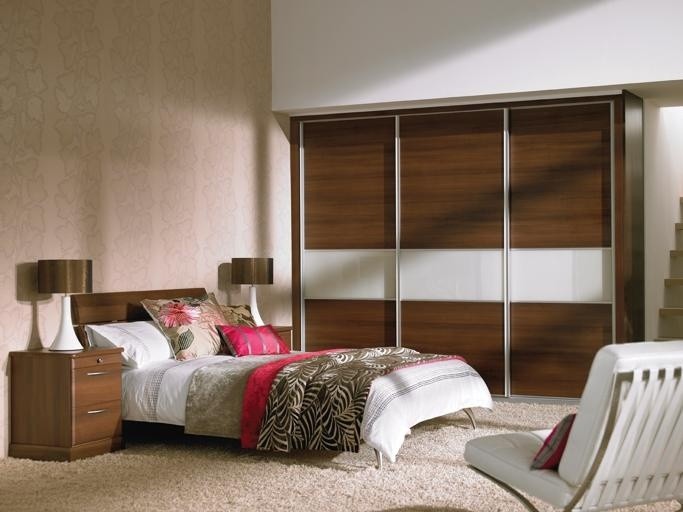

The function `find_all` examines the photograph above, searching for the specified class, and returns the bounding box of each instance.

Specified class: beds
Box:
[71,288,476,469]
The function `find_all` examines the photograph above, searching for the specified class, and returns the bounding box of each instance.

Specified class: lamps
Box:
[231,258,273,326]
[37,259,92,351]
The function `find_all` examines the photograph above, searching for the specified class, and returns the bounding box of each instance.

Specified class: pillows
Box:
[530,412,576,470]
[84,291,290,369]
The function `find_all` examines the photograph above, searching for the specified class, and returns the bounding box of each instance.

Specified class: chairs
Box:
[464,340,683,512]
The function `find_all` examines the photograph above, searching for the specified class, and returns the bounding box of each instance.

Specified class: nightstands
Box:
[9,347,125,463]
